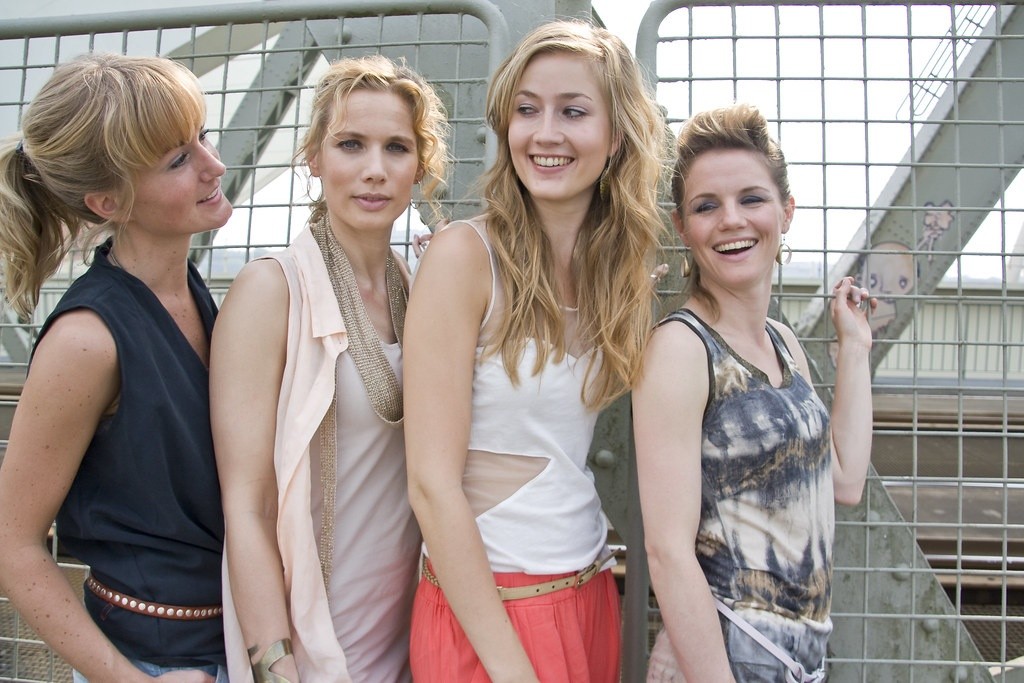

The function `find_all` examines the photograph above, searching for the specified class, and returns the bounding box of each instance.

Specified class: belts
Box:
[712,597,827,683]
[422,548,623,601]
[86,574,224,621]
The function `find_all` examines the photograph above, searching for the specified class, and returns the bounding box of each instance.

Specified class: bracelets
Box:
[247,639,294,683]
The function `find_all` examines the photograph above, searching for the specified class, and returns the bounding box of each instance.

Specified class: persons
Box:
[404,22,661,683]
[0,53,233,683]
[631,107,877,683]
[207,58,444,683]
